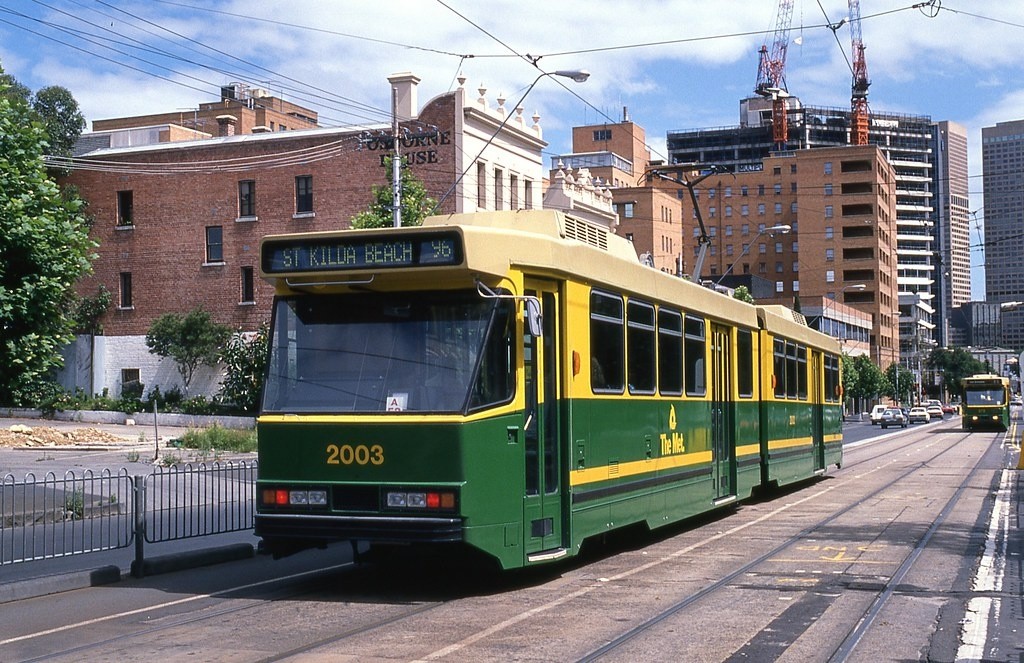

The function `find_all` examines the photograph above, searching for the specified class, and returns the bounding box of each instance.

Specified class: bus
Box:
[959,372,1011,432]
[252,161,843,571]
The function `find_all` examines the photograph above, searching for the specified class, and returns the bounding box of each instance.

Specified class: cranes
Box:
[753,0,794,152]
[848,0,872,146]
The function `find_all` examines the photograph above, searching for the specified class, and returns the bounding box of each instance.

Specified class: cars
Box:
[869,399,959,428]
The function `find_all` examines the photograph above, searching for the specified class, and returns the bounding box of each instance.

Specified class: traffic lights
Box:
[915,392,919,406]
